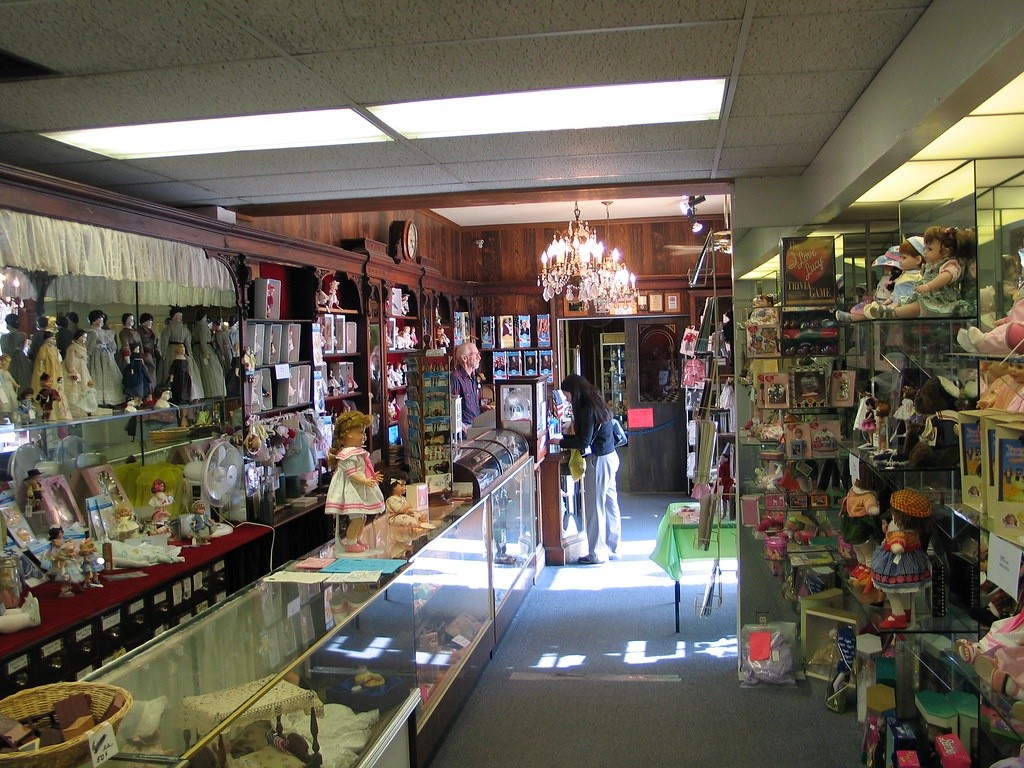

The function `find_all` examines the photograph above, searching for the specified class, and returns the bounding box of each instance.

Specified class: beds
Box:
[180,673,323,768]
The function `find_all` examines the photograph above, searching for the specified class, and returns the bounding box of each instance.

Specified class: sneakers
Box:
[578,556,592,564]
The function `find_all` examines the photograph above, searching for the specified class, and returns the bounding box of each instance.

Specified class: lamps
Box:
[679,195,709,233]
[536,201,639,305]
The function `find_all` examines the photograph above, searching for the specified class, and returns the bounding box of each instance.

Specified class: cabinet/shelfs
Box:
[600,332,627,431]
[316,306,363,402]
[738,301,1024,768]
[383,311,420,428]
[687,289,738,500]
[246,318,312,414]
[480,347,554,387]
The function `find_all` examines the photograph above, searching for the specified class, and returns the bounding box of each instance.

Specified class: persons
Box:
[435,307,478,348]
[743,294,778,324]
[713,453,734,519]
[835,226,1024,457]
[483,317,551,412]
[605,349,627,415]
[547,374,619,564]
[491,487,517,563]
[0,280,427,598]
[967,447,1024,528]
[839,466,933,629]
[450,342,495,439]
[832,621,856,710]
[957,606,1024,721]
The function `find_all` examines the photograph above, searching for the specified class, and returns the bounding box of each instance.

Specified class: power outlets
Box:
[756,610,770,623]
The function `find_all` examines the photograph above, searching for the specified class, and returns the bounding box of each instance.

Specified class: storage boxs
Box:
[887,717,916,768]
[800,588,843,665]
[806,607,861,688]
[455,311,554,384]
[775,235,836,304]
[936,733,972,768]
[895,749,920,768]
[39,278,357,535]
[914,688,959,768]
[946,689,979,768]
[405,482,429,511]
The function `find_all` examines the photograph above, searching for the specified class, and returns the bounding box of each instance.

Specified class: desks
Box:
[650,500,740,632]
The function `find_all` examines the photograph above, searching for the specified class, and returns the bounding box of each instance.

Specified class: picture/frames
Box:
[563,293,590,319]
[595,304,609,313]
[665,289,684,316]
[647,292,665,315]
[638,294,648,312]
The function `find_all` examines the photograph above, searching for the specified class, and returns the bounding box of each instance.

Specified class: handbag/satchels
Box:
[579,444,592,457]
[610,418,627,447]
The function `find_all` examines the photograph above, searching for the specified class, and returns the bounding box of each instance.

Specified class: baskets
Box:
[1,681,131,768]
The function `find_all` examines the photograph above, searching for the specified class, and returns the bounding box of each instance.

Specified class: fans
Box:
[183,439,246,538]
[7,444,63,475]
[58,436,105,472]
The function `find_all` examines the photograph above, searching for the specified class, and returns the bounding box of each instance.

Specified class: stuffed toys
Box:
[872,376,979,471]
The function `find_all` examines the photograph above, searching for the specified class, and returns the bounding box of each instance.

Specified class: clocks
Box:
[402,218,418,262]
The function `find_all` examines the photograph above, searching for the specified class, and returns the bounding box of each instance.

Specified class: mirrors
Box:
[0,274,241,541]
[762,156,1024,716]
